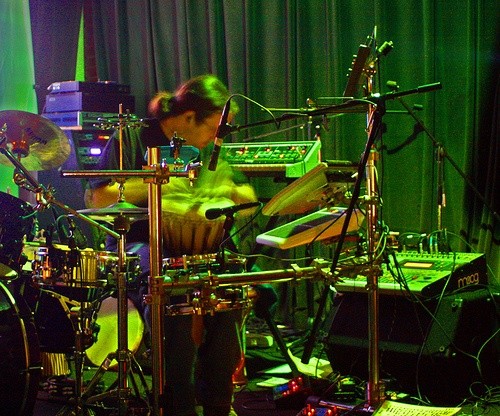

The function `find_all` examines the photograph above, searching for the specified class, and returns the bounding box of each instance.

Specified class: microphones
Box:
[205,202,261,220]
[208,101,231,171]
[343,45,370,103]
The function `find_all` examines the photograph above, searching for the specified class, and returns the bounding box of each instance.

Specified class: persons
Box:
[84,75,260,416]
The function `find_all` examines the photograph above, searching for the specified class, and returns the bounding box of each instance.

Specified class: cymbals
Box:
[67,203,148,224]
[50,374,105,397]
[0,110,70,171]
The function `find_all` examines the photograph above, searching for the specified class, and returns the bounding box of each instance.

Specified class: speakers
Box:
[326,286,500,407]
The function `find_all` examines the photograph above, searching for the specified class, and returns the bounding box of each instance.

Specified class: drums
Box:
[0,190,35,281]
[84,296,145,370]
[0,281,42,416]
[160,192,236,256]
[33,246,143,290]
[162,254,252,316]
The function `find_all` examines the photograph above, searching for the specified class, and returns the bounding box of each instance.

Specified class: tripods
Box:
[68,215,155,416]
